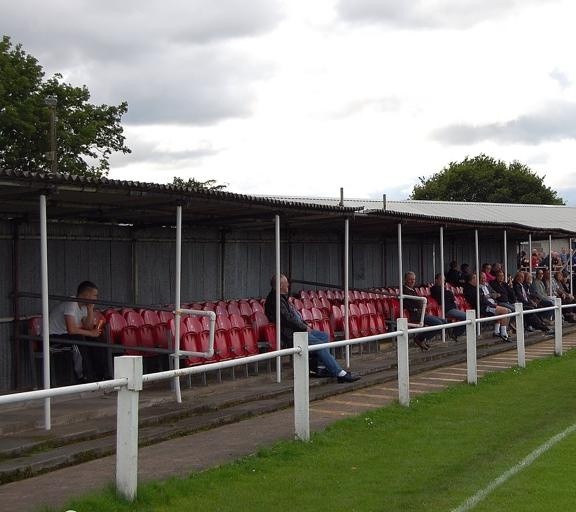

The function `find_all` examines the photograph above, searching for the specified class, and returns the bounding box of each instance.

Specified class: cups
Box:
[93,320,104,329]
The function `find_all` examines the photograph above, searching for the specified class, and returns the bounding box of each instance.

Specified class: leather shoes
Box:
[526,327,534,332]
[415,337,428,350]
[451,336,457,342]
[416,335,431,348]
[336,372,360,383]
[310,367,333,378]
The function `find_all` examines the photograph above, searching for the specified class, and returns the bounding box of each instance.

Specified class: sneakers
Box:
[493,332,511,338]
[544,329,555,337]
[500,335,512,344]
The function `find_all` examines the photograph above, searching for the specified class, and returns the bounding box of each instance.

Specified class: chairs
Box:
[25,283,505,390]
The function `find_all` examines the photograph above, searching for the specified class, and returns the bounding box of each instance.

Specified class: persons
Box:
[264,273,361,384]
[38,280,103,384]
[446,247,576,343]
[402,270,446,350]
[430,272,466,342]
